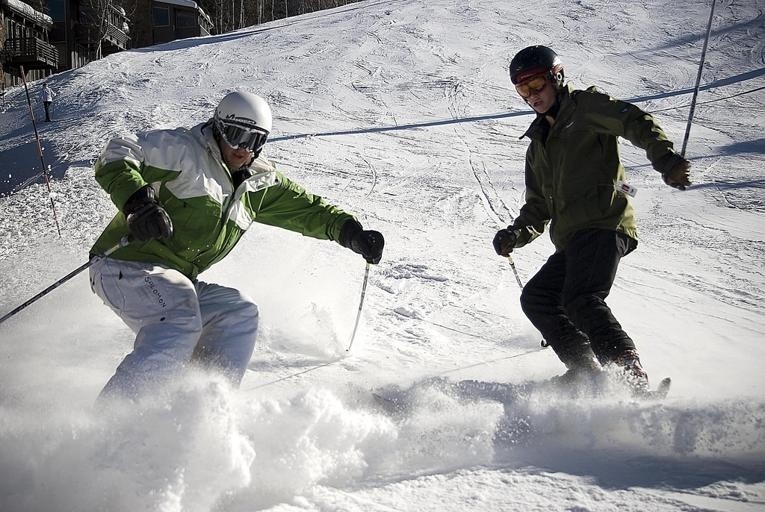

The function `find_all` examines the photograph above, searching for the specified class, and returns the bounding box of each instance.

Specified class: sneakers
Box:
[608,363,650,386]
[547,358,602,384]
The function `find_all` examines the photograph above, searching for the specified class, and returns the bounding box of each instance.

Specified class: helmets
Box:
[508,45,565,82]
[215,91,273,134]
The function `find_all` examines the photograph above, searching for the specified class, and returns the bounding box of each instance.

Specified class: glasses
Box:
[513,71,551,99]
[215,119,267,152]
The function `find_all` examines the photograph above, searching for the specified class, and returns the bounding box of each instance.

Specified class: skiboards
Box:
[371,378,672,432]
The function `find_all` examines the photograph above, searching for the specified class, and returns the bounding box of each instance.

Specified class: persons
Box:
[83,89,387,402]
[490,44,695,400]
[36,82,57,122]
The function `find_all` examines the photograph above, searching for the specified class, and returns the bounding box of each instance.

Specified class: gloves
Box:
[122,185,174,241]
[493,228,516,258]
[340,216,384,265]
[652,149,693,190]
